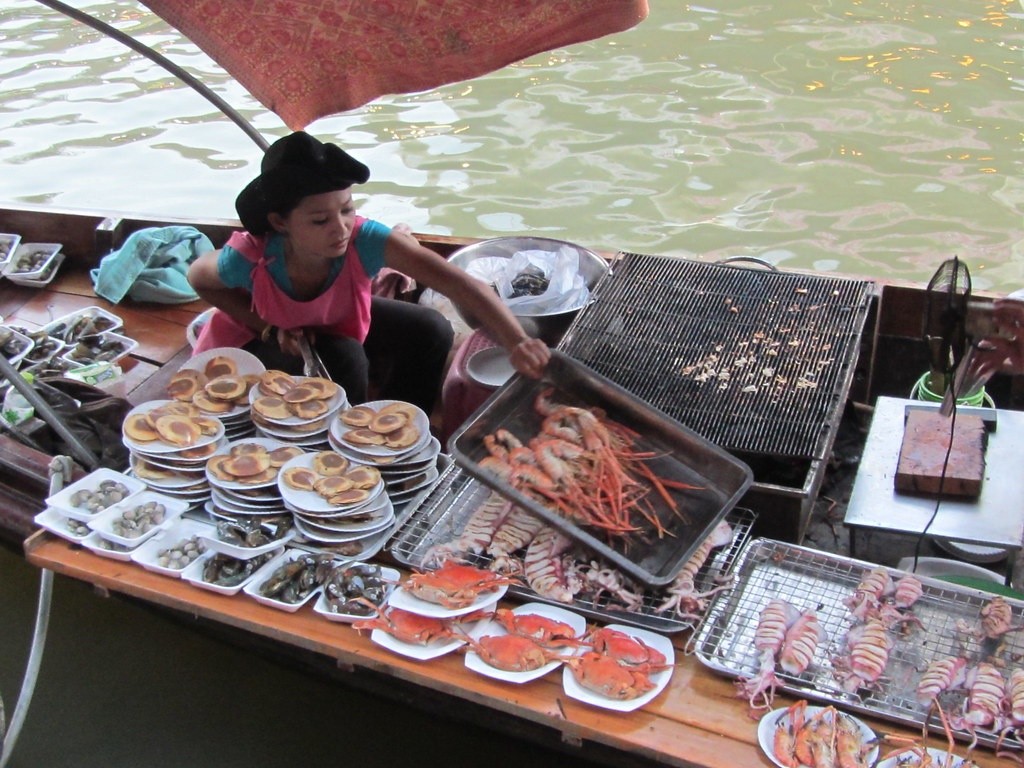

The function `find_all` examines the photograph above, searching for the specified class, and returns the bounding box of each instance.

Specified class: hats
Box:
[234,127,370,236]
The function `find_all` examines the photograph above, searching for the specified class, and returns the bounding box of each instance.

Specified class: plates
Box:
[758,706,879,768]
[879,745,980,766]
[369,566,676,712]
[121,345,442,542]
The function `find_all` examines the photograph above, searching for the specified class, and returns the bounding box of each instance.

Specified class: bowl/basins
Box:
[33,466,401,630]
[438,237,611,343]
[0,231,139,392]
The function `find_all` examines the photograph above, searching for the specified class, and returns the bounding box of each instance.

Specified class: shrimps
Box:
[480,388,707,556]
[773,699,978,768]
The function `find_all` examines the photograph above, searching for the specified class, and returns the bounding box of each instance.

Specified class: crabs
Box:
[346,562,685,701]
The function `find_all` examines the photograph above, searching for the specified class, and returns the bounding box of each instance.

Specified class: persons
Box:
[187,131,552,417]
